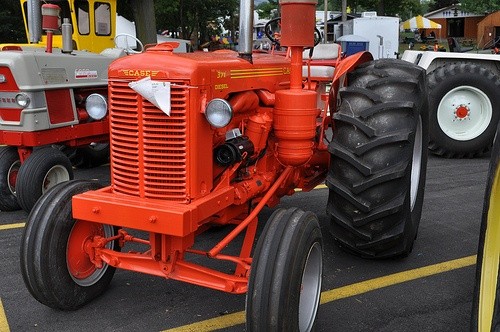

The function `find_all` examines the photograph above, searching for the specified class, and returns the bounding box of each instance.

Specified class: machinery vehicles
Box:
[0,0,158,213]
[18,0,431,332]
[400,50,500,159]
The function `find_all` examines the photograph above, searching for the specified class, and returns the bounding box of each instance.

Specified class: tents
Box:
[400,15,442,46]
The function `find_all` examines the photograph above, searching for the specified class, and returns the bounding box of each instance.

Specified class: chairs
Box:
[301,43,342,99]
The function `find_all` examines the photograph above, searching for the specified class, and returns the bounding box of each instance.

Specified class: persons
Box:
[264,41,271,50]
[447,38,456,51]
[420,31,425,43]
[430,31,435,37]
